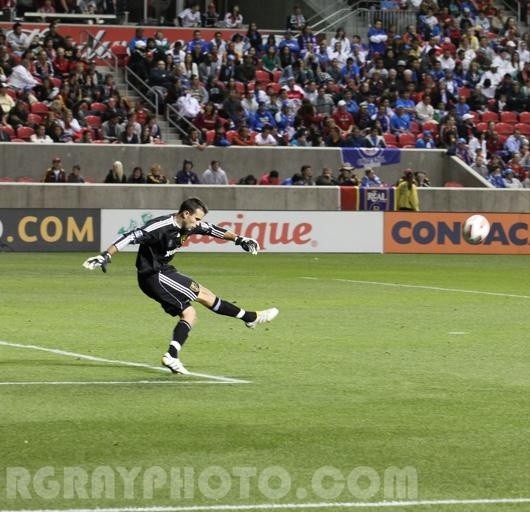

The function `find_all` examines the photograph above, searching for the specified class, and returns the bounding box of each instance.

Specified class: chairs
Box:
[224,131,238,145]
[0,126,16,141]
[382,132,396,144]
[205,131,215,144]
[515,123,530,134]
[16,127,35,140]
[255,70,270,82]
[416,132,424,140]
[467,111,482,123]
[51,79,61,86]
[486,100,497,110]
[402,143,416,148]
[475,122,488,132]
[423,119,441,137]
[76,128,96,142]
[85,115,101,129]
[482,112,501,122]
[398,131,416,147]
[91,102,107,114]
[234,81,246,98]
[268,82,280,95]
[6,88,18,100]
[386,143,398,148]
[444,182,464,188]
[408,122,420,134]
[16,176,31,182]
[248,82,257,93]
[274,71,282,83]
[0,176,16,183]
[258,81,268,92]
[519,110,530,123]
[111,44,129,66]
[9,139,25,144]
[31,101,48,115]
[494,123,514,134]
[458,88,470,99]
[92,138,110,144]
[27,113,43,126]
[499,110,517,123]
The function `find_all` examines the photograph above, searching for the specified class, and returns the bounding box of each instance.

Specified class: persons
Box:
[41,157,66,183]
[318,166,337,185]
[176,159,200,183]
[395,169,420,211]
[337,163,358,186]
[147,163,170,184]
[367,56,389,151]
[0,0,107,24]
[175,4,201,26]
[103,161,127,182]
[390,41,440,148]
[286,5,306,30]
[115,27,367,149]
[67,165,84,182]
[82,197,279,374]
[368,21,387,55]
[204,3,220,27]
[441,33,530,116]
[225,6,243,27]
[345,0,520,38]
[439,113,530,187]
[127,167,144,183]
[201,160,228,184]
[238,174,257,185]
[0,23,114,144]
[258,170,282,185]
[360,166,385,187]
[301,165,316,185]
[282,173,304,185]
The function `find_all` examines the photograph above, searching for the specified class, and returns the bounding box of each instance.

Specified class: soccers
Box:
[462,214,491,246]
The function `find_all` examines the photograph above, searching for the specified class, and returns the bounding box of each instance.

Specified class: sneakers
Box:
[163,353,190,375]
[247,308,280,327]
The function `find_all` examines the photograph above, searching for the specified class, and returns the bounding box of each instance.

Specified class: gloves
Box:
[235,235,260,256]
[82,251,113,273]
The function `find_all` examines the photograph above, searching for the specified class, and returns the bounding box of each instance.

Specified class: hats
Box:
[52,156,62,163]
[463,113,476,120]
[425,131,432,137]
[503,169,512,175]
[395,60,408,67]
[281,101,294,109]
[338,162,355,172]
[404,168,414,174]
[338,100,348,107]
[242,50,254,59]
